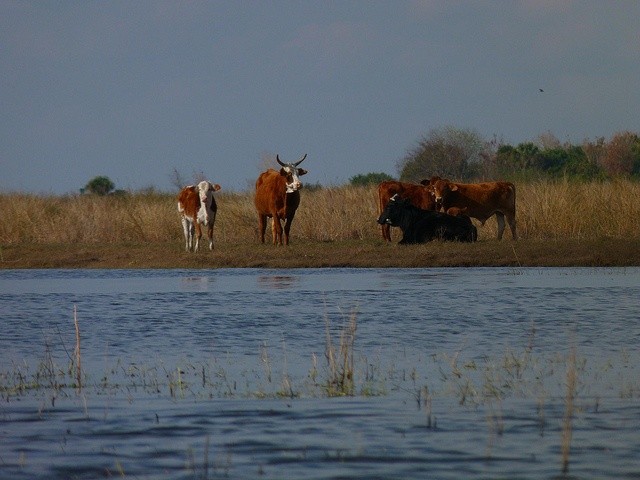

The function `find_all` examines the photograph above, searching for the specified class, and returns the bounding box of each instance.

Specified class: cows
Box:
[431,178,520,242]
[376,174,450,243]
[253,153,309,250]
[376,192,478,246]
[176,179,222,254]
